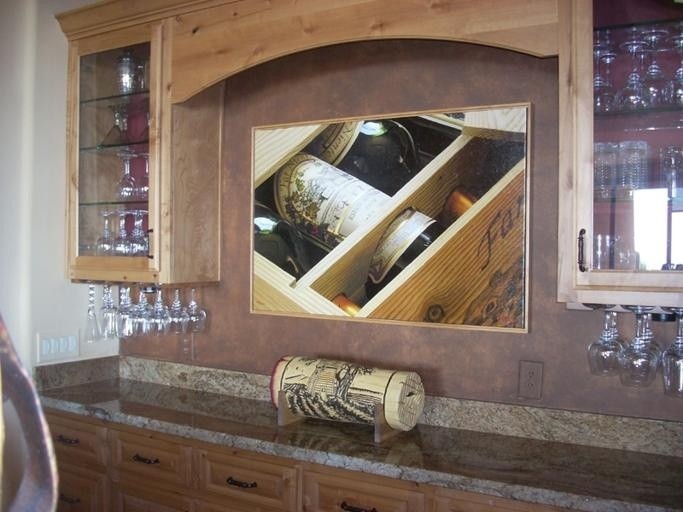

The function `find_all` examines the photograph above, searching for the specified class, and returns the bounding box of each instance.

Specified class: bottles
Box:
[260,156,444,281]
[348,122,477,224]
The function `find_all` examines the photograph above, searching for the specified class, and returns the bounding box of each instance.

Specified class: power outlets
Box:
[516,359,542,399]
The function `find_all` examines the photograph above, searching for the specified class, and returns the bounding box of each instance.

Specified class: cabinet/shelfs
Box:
[556,1,683,308]
[66,16,221,284]
[39,404,568,512]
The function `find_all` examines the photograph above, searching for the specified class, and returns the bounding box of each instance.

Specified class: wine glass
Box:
[583,304,683,397]
[82,282,207,343]
[591,29,682,113]
[96,152,147,254]
[100,102,138,147]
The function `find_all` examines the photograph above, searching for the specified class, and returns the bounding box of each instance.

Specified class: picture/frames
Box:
[247,101,533,335]
